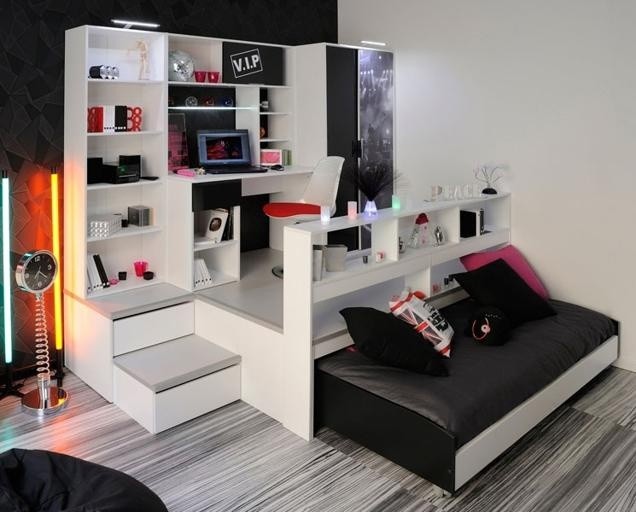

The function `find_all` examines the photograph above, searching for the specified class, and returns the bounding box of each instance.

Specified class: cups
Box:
[207,71,220,83]
[195,71,207,85]
[134,261,147,277]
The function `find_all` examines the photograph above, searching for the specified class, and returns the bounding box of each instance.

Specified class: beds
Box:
[283,188,620,494]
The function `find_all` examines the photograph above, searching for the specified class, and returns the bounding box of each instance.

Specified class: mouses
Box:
[271,165,284,171]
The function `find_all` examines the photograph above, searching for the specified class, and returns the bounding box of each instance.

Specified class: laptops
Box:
[196,128,268,174]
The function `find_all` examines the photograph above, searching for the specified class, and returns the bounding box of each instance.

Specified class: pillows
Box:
[338,307,457,377]
[460,244,549,299]
[388,290,455,358]
[452,258,557,332]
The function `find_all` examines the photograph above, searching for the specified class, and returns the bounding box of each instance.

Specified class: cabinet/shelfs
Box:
[64,24,395,436]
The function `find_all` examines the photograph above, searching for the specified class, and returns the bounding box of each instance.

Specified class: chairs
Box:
[263,157,345,279]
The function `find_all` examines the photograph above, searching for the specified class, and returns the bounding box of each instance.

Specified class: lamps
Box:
[51,165,65,387]
[361,40,386,46]
[0,167,25,401]
[110,18,160,29]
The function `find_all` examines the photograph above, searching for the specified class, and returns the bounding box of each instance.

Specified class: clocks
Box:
[11,249,58,293]
